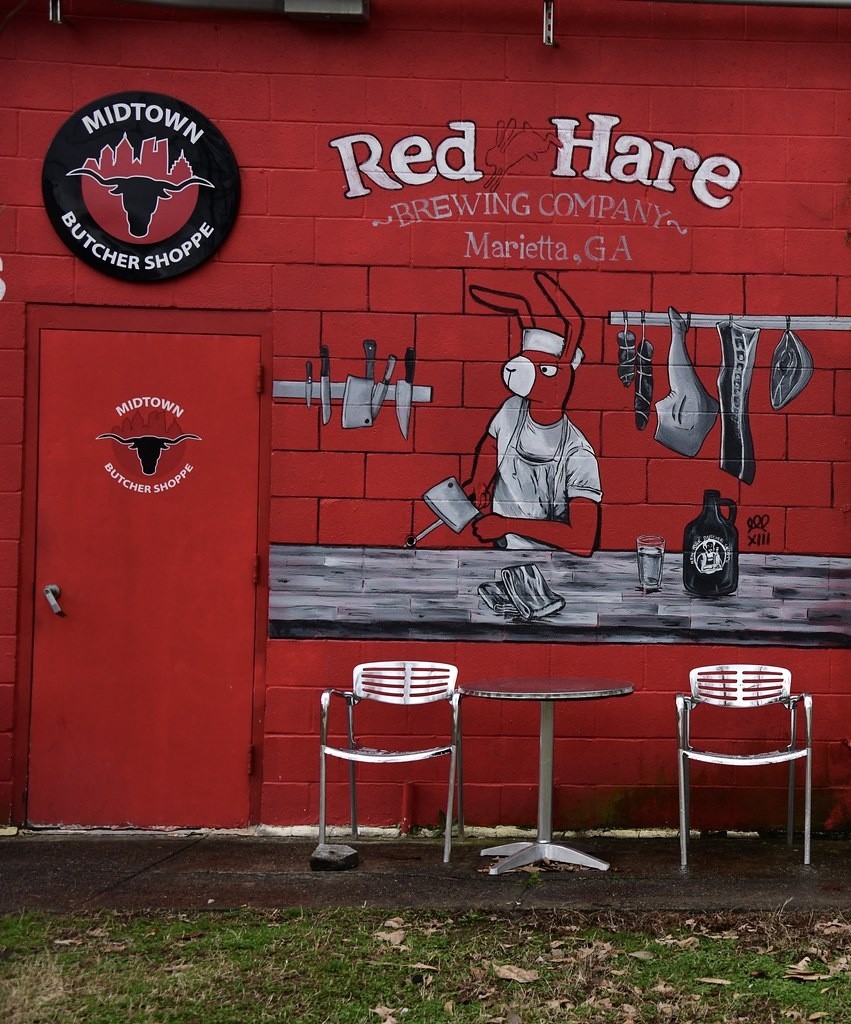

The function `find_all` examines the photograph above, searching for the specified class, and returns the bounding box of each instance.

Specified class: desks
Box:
[457,676,635,876]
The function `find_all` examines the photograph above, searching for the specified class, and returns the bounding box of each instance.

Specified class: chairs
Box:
[316,660,467,864]
[675,663,814,867]
